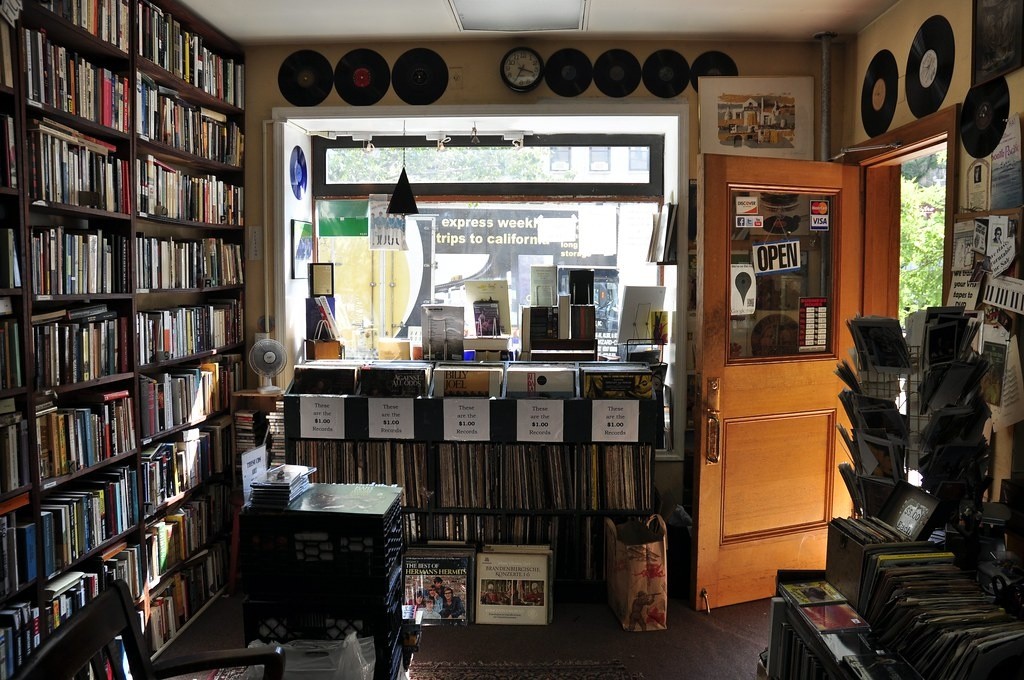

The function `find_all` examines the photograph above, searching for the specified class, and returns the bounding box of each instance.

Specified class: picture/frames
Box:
[696,74,816,163]
[968,0,1024,93]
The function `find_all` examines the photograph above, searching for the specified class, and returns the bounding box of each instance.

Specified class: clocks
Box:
[500,46,544,91]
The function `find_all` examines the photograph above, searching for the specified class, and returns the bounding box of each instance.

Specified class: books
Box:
[238,400,287,486]
[762,528,1024,680]
[292,438,657,627]
[827,304,996,524]
[0,210,249,417]
[291,266,668,396]
[0,0,248,210]
[0,417,234,680]
[647,202,679,265]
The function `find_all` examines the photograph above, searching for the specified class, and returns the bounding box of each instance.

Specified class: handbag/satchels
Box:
[606,513,668,631]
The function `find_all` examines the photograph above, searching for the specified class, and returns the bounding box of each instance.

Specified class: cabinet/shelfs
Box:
[0,0,253,677]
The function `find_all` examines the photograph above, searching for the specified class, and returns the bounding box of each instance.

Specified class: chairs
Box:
[8,577,287,680]
